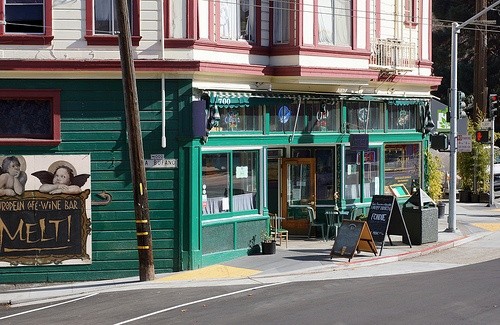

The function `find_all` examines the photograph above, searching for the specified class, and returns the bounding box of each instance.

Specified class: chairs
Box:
[326,209,341,240]
[269,213,289,248]
[306,206,325,241]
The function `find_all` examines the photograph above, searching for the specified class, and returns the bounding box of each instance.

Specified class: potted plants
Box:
[423,147,446,218]
[457,102,500,203]
[261,231,276,254]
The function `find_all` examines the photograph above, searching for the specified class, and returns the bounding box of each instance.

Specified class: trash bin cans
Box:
[403,188,438,244]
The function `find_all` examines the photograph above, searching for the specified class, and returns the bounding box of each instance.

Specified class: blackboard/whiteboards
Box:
[367,195,408,242]
[332,220,377,257]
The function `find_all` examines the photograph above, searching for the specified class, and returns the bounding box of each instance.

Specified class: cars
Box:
[472,145,500,191]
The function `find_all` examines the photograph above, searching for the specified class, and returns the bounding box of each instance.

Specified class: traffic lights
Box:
[458,90,468,119]
[476,130,489,143]
[488,93,499,119]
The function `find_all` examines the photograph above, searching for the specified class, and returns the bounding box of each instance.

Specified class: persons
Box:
[39,165,80,195]
[0,156,26,196]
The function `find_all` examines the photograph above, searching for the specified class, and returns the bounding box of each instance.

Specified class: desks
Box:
[325,211,349,224]
[269,216,286,227]
